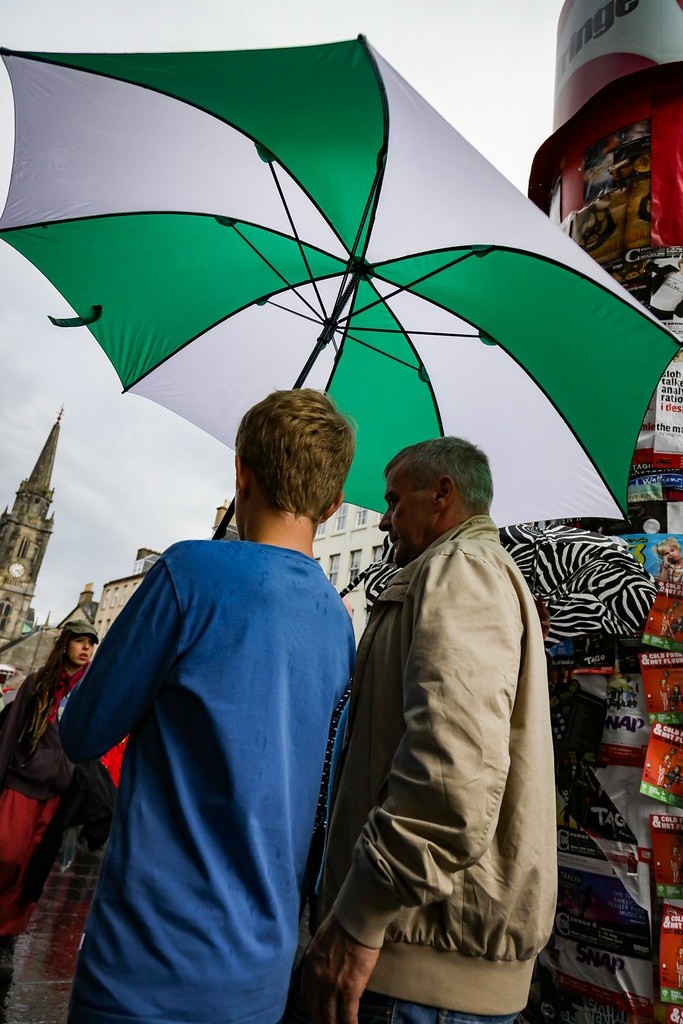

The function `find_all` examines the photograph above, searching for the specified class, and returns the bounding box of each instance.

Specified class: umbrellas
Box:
[0,34,683,540]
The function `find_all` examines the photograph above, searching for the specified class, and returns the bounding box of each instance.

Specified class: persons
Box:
[58,388,357,1024]
[303,436,558,1024]
[0,619,129,976]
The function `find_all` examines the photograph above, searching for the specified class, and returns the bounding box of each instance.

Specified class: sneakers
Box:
[0,936,14,974]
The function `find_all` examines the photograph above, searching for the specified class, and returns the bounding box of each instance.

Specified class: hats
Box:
[63,620,99,644]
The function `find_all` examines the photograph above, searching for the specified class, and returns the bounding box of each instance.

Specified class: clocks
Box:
[7,561,26,579]
